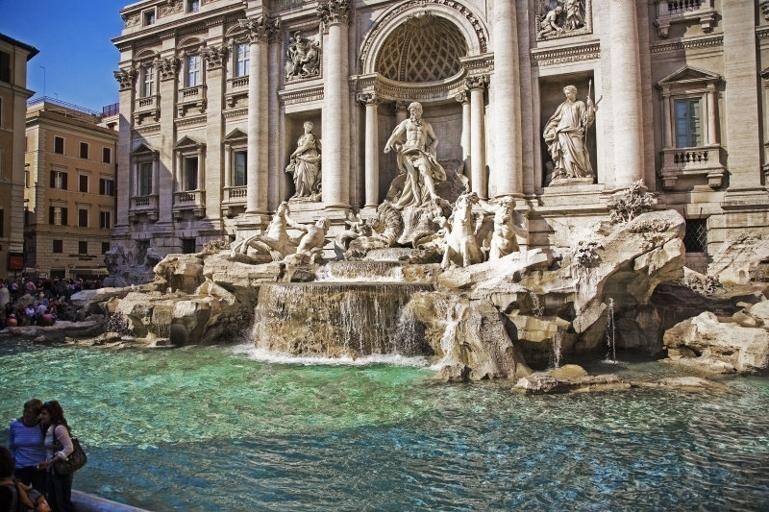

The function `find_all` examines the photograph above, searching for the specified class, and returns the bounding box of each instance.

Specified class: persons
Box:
[37,400,74,512]
[288,35,320,78]
[0,276,83,326]
[0,445,24,512]
[489,195,528,259]
[384,102,440,206]
[537,5,565,38]
[9,399,47,498]
[291,122,318,198]
[285,208,330,264]
[543,85,595,177]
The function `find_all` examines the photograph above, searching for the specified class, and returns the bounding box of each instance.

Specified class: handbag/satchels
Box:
[53,423,88,472]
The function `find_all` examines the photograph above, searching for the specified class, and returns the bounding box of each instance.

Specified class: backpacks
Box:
[0,479,51,512]
